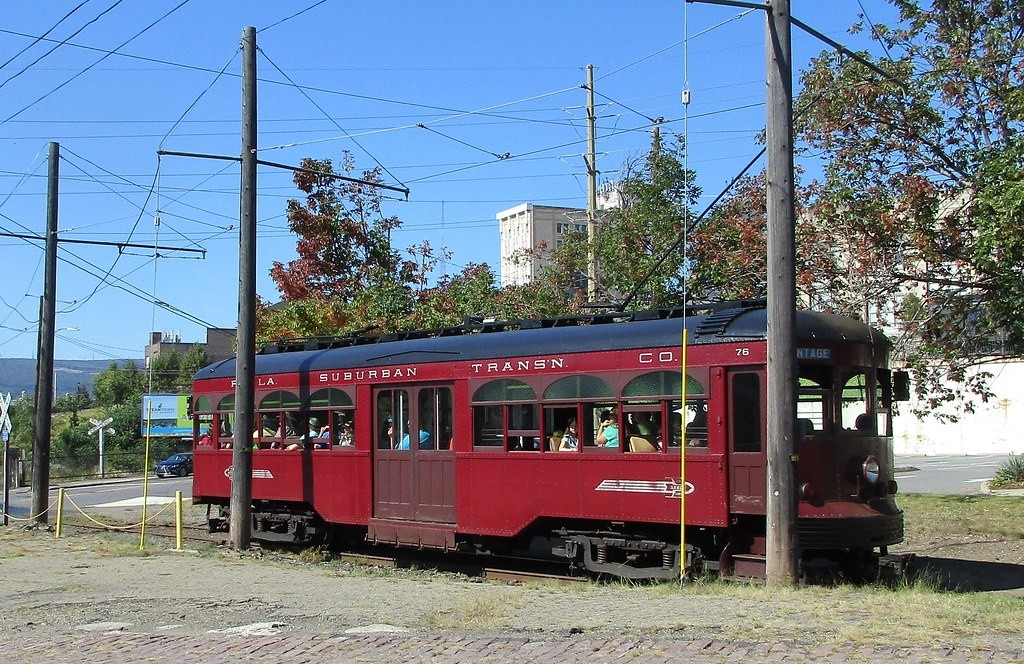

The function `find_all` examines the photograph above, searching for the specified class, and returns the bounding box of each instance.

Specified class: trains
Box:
[193,299,910,585]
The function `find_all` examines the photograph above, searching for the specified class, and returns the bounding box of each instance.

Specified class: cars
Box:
[157,453,193,478]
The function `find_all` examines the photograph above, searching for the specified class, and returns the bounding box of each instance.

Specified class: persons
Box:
[200,420,234,449]
[269,417,354,451]
[387,418,433,450]
[559,416,578,452]
[596,407,661,448]
[252,416,276,449]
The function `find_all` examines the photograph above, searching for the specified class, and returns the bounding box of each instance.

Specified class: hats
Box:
[309,418,320,431]
[276,411,296,421]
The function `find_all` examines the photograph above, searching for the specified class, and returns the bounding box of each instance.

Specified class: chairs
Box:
[626,434,661,453]
[545,435,562,452]
